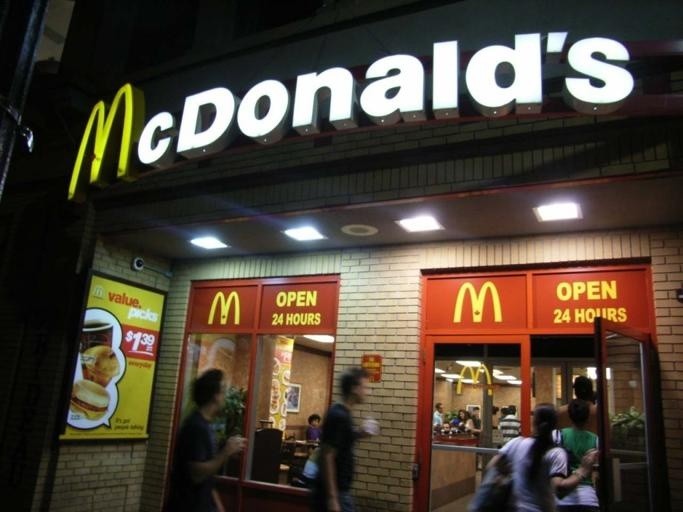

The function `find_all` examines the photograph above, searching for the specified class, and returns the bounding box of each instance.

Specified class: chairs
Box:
[260,419,319,476]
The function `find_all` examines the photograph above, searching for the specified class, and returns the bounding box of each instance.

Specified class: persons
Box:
[160,368,249,511]
[306,368,379,511]
[432,372,601,512]
[302,413,322,484]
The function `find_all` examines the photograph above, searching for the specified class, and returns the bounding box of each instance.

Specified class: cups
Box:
[78,319,114,384]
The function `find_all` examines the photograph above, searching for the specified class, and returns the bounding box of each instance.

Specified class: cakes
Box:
[82,346,120,390]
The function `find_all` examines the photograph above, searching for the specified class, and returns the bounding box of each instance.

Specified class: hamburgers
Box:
[70,379,108,423]
